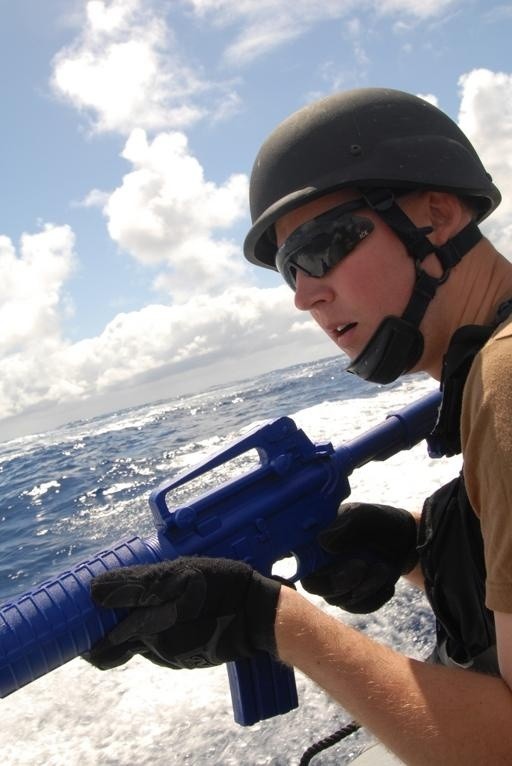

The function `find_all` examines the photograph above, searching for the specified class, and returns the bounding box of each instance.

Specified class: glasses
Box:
[275,192,376,292]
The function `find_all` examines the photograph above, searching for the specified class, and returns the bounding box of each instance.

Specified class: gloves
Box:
[293,502,418,616]
[82,555,281,673]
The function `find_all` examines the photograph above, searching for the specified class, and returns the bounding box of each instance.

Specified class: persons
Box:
[70,84,512,765]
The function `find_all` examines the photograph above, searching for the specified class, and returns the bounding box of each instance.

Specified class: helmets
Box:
[243,88,502,270]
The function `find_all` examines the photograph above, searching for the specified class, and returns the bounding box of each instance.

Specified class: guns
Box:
[0,390,445,725]
